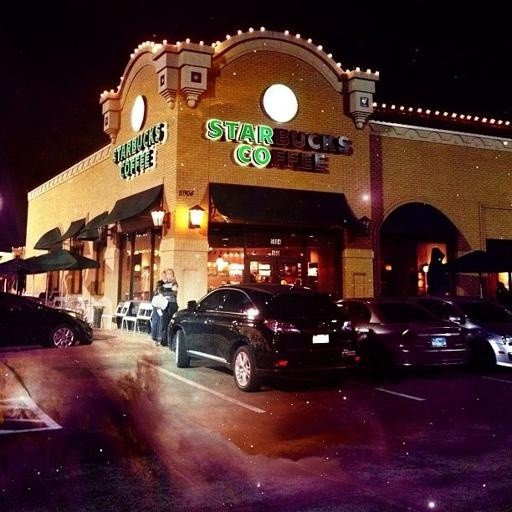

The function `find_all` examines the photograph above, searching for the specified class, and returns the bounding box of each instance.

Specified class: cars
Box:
[1,291,94,348]
[335,296,474,374]
[415,294,512,375]
[167,281,362,393]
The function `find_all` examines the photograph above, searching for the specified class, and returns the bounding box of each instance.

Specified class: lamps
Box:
[187,204,206,230]
[148,203,167,232]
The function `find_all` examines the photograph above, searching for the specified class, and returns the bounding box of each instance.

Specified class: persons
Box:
[150,280,166,346]
[496,282,512,307]
[159,269,179,346]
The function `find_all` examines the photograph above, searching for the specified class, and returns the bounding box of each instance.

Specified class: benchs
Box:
[100,301,154,336]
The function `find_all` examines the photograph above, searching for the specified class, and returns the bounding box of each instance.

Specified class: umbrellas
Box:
[0,248,101,308]
[440,250,512,299]
